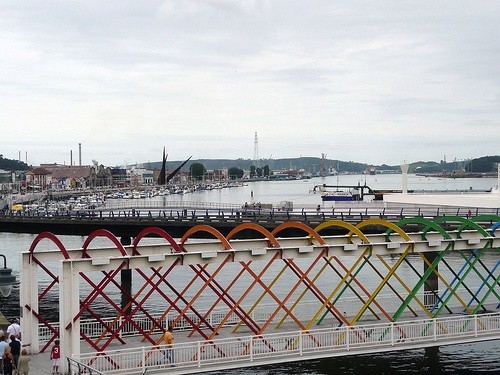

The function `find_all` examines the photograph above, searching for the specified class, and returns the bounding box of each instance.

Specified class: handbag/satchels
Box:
[15,333,20,337]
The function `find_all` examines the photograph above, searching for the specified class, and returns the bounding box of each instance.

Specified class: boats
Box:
[6,182,249,215]
[321,185,355,202]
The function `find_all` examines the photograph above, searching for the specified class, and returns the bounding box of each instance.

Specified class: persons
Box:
[7,319,21,340]
[50,340,60,374]
[0,330,31,375]
[156,326,175,367]
